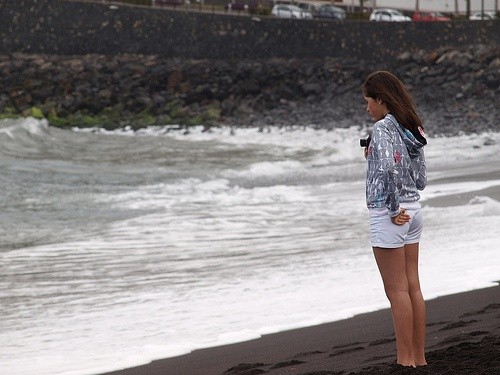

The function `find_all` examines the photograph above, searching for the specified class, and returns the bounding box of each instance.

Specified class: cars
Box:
[369,8,413,21]
[318,4,346,19]
[224,0,259,14]
[469,11,494,20]
[272,4,313,19]
[413,10,451,21]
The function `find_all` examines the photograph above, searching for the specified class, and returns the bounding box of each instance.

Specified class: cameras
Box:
[360,136,372,151]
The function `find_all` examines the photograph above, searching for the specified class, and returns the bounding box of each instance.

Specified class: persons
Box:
[363,71,428,371]
